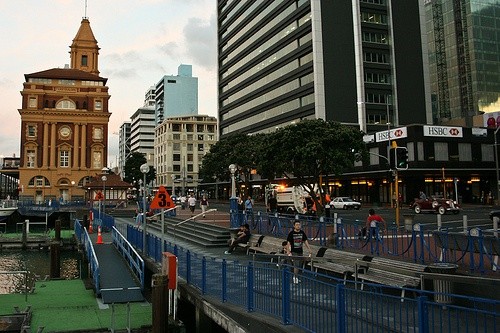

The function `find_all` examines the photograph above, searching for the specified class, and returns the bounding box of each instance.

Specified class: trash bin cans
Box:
[428,263,459,305]
[402,214,415,233]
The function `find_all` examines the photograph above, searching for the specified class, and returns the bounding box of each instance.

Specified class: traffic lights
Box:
[389,147,396,170]
[396,147,408,169]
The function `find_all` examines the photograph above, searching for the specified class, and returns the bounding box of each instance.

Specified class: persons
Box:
[133,209,157,229]
[267,194,278,212]
[420,191,426,200]
[392,191,404,208]
[357,209,387,240]
[276,220,312,284]
[480,189,495,203]
[238,195,255,227]
[179,192,196,217]
[225,224,250,254]
[200,194,209,218]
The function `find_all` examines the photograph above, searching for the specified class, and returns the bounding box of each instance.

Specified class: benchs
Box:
[228,233,426,302]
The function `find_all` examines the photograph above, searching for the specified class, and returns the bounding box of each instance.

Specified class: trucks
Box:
[276,186,331,215]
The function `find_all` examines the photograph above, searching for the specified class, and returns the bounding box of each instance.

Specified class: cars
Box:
[408,194,462,215]
[330,197,361,211]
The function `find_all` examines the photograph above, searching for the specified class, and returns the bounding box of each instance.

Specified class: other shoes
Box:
[293,277,298,284]
[224,250,231,254]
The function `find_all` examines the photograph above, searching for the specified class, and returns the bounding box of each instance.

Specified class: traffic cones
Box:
[88,224,93,234]
[96,225,104,244]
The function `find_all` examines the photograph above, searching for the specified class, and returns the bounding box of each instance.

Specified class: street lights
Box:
[228,163,240,228]
[139,164,149,231]
[171,174,176,194]
[138,179,142,196]
[102,176,107,218]
